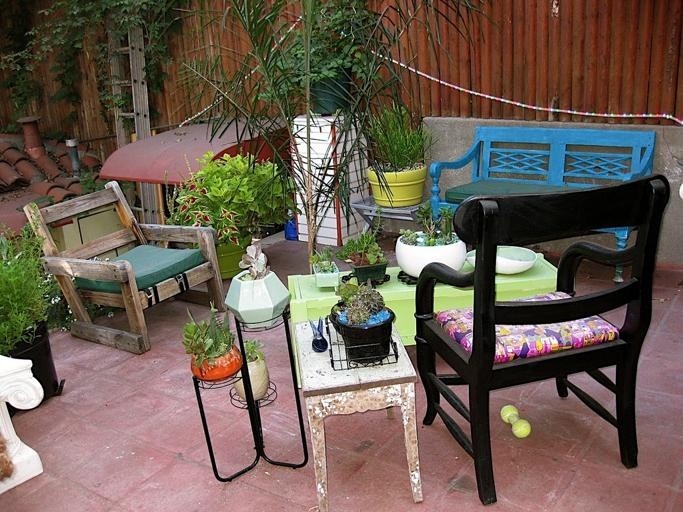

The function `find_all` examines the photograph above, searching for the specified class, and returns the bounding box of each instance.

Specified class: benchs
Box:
[429,125,656,286]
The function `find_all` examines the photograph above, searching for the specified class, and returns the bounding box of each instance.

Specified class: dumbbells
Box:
[501,405,530,439]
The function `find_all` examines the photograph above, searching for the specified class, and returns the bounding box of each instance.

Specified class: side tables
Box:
[295,321,424,512]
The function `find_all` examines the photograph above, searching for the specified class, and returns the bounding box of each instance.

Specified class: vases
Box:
[468,244,537,275]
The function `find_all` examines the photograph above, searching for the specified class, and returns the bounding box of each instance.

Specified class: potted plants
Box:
[181,301,243,382]
[164,152,302,279]
[366,102,427,207]
[334,234,391,288]
[255,1,382,116]
[0,203,65,417]
[329,284,396,363]
[224,239,291,329]
[395,201,467,284]
[234,339,270,402]
[310,247,340,292]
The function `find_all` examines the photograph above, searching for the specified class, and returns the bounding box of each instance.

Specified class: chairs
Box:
[414,174,670,505]
[23,181,225,355]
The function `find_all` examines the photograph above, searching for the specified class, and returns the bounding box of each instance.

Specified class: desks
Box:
[350,194,431,239]
[288,252,558,389]
